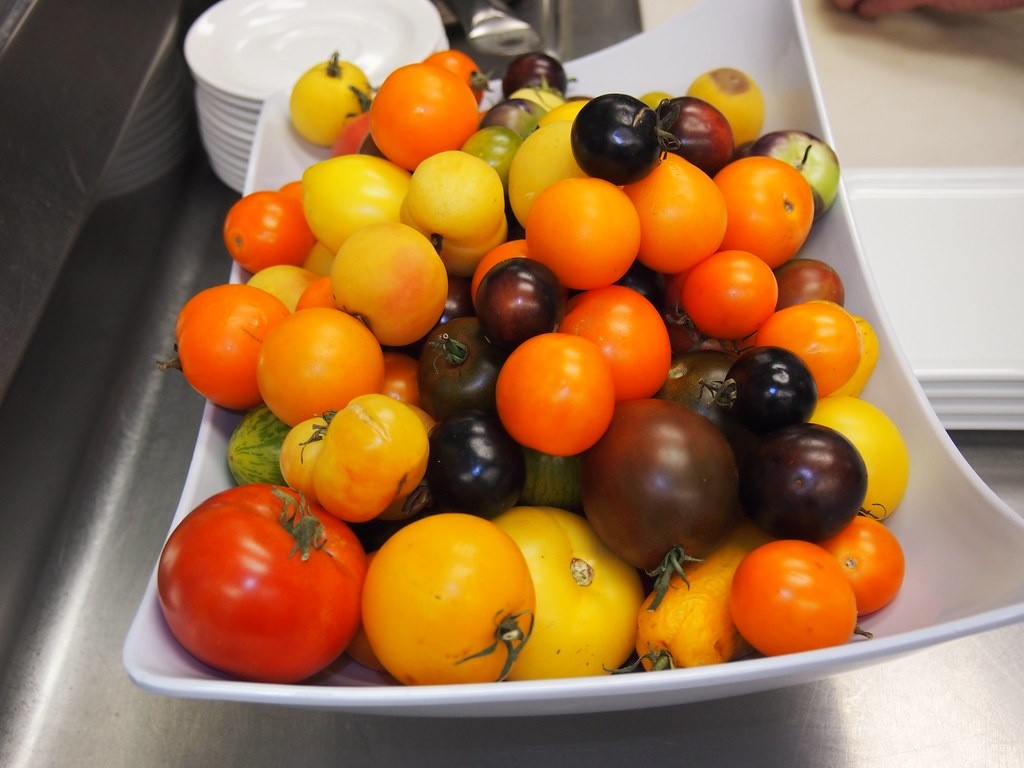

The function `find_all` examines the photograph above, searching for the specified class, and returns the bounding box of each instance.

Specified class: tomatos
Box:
[156,51,910,688]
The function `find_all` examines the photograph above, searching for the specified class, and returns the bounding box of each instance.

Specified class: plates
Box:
[123,0,1024,714]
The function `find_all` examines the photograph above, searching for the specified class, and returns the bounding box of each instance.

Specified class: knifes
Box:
[443,0,542,78]
